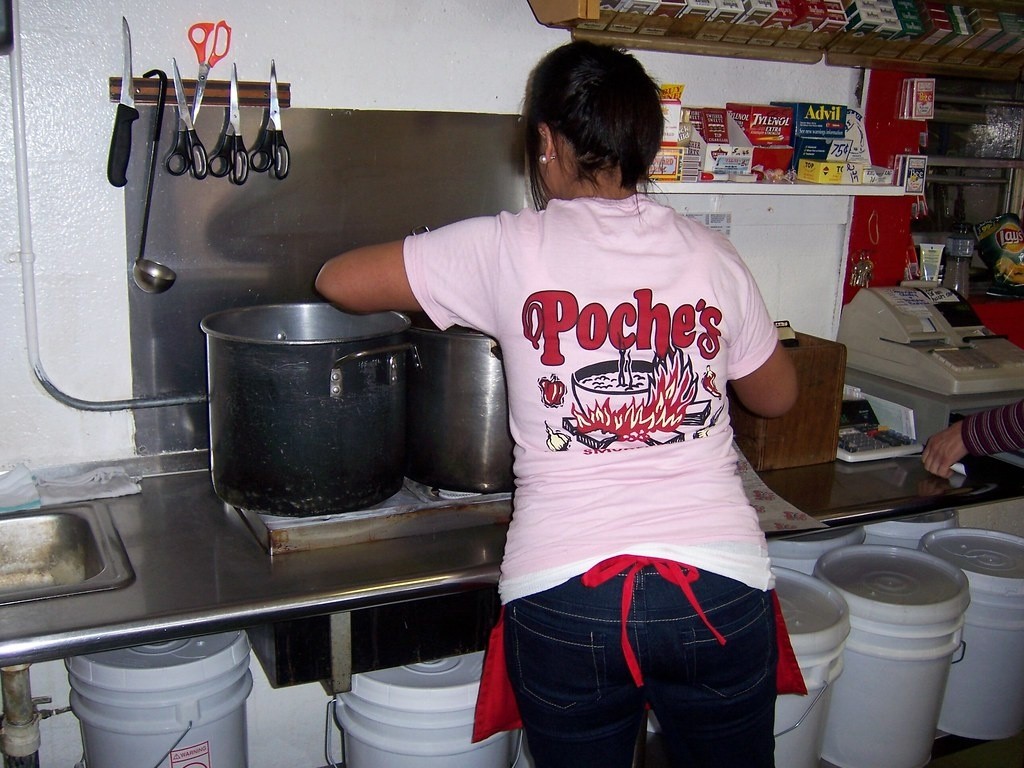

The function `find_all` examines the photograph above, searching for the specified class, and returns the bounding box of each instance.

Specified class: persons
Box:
[312,41,801,768]
[920,399,1024,479]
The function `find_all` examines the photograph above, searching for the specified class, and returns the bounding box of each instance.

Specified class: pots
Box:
[408,311,516,493]
[572,357,658,421]
[196,301,415,519]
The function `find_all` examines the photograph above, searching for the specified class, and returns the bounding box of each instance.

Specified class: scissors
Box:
[187,20,233,126]
[209,63,249,186]
[165,58,210,181]
[247,60,291,180]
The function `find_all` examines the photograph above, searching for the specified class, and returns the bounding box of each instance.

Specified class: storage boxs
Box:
[791,136,854,175]
[728,330,847,473]
[771,100,848,137]
[680,108,754,175]
[726,101,794,144]
[751,144,794,181]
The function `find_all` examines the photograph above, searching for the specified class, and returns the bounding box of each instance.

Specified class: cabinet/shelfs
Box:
[527,0,839,65]
[825,28,1024,81]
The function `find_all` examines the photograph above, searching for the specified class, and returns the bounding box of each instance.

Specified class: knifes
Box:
[102,16,141,188]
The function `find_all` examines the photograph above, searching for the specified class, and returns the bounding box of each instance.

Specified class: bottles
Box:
[942,222,976,301]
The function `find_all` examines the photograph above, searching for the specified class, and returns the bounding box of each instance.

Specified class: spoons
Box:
[129,69,178,293]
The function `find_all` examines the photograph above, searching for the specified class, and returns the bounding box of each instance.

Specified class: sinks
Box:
[0,502,137,607]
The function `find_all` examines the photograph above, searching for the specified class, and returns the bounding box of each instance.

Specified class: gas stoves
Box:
[219,475,515,557]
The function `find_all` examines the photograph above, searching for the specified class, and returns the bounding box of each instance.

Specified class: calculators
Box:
[836,397,924,464]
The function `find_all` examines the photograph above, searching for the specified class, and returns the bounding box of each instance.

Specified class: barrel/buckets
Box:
[919,524,1023,742]
[860,508,962,550]
[810,543,970,768]
[323,650,522,768]
[764,524,867,576]
[768,563,851,768]
[64,628,253,768]
